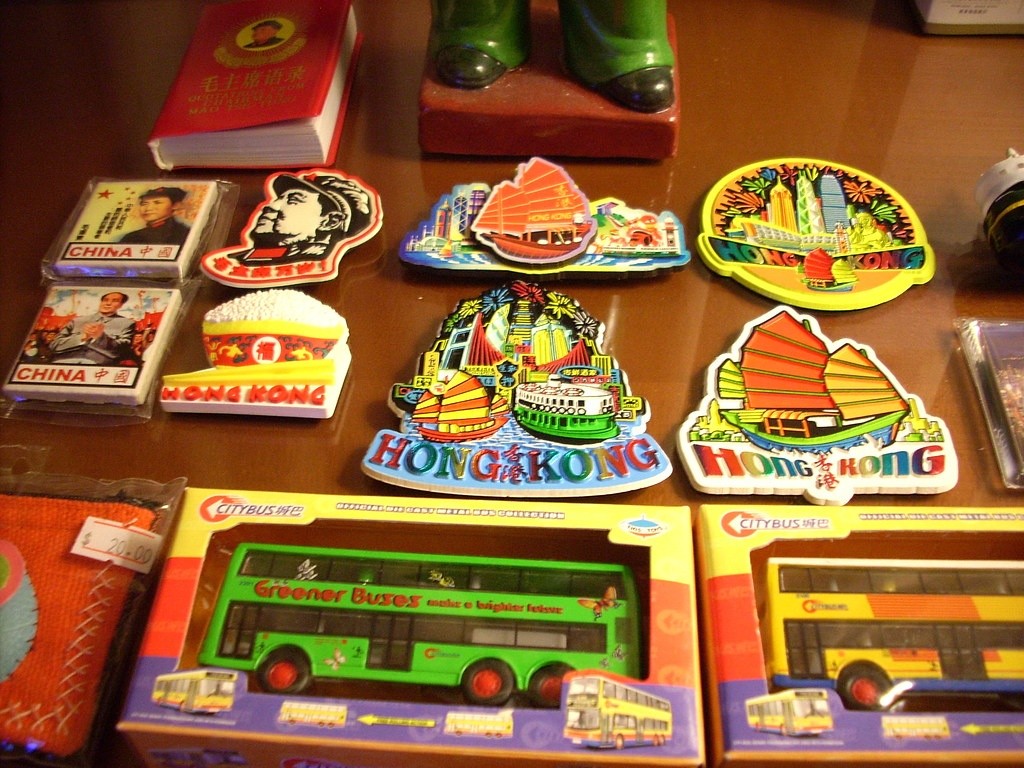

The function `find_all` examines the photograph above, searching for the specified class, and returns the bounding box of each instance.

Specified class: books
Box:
[146,0,364,172]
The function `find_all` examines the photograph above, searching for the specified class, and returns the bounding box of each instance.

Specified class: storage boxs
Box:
[4,286,183,408]
[914,0,1024,36]
[693,502,1024,768]
[113,485,706,768]
[54,181,219,281]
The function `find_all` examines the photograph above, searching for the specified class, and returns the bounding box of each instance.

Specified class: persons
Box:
[427,0,676,114]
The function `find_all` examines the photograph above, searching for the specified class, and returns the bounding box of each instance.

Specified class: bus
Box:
[194,540,644,707]
[750,558,1024,714]
